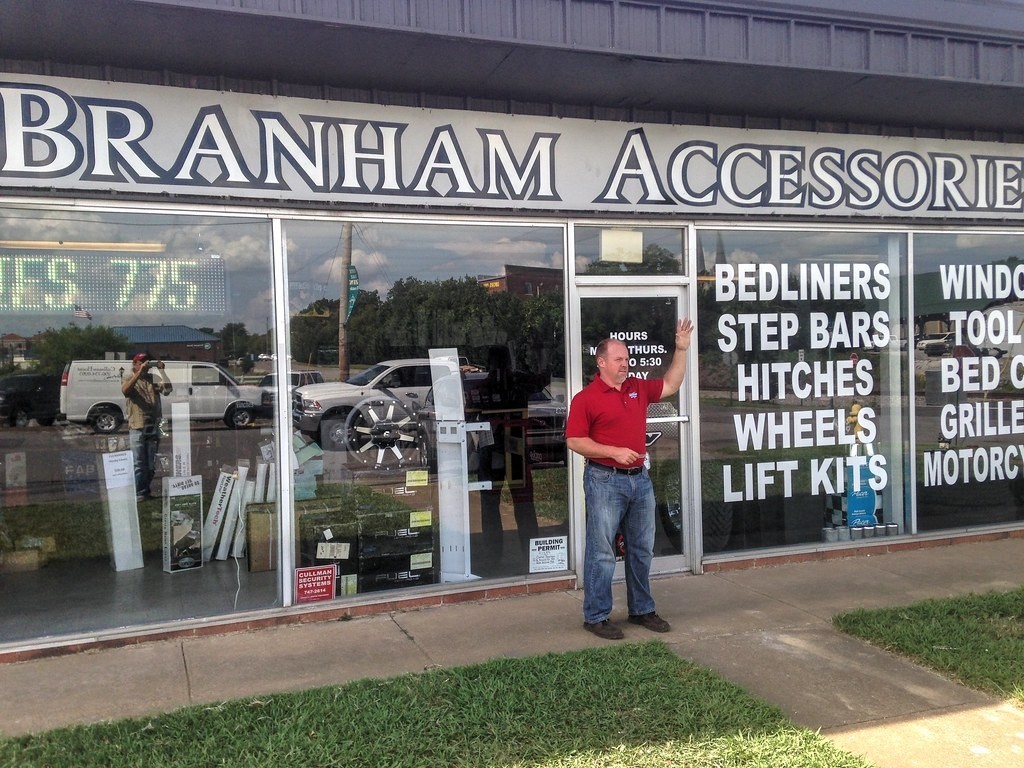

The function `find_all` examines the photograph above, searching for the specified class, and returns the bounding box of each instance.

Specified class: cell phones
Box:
[148,362,158,367]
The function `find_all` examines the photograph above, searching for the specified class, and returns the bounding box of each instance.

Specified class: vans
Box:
[60,360,270,434]
[258,370,325,416]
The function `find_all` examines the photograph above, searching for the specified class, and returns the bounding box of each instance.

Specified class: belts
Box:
[585,459,643,475]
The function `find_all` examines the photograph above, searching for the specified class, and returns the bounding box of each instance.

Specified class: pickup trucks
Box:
[292,358,495,452]
[876,336,909,350]
[433,357,486,375]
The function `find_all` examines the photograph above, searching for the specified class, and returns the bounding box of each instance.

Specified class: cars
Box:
[258,353,275,359]
[917,332,957,350]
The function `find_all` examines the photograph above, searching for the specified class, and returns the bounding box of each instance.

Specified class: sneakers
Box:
[628,611,670,631]
[583,618,624,640]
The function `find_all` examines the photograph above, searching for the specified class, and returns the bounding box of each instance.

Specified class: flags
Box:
[76,305,93,321]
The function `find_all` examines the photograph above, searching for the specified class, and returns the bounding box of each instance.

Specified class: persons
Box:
[566,318,694,639]
[121,354,173,502]
[472,337,551,571]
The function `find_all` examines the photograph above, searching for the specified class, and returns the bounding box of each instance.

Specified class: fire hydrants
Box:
[846,402,867,441]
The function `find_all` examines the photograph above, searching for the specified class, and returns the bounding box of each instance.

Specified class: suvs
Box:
[0,373,63,427]
[418,373,569,477]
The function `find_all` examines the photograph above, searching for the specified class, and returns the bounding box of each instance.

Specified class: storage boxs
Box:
[246,497,344,573]
[345,551,435,592]
[313,481,372,499]
[298,511,358,596]
[341,491,434,560]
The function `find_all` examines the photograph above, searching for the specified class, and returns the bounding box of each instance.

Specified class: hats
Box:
[133,353,147,362]
